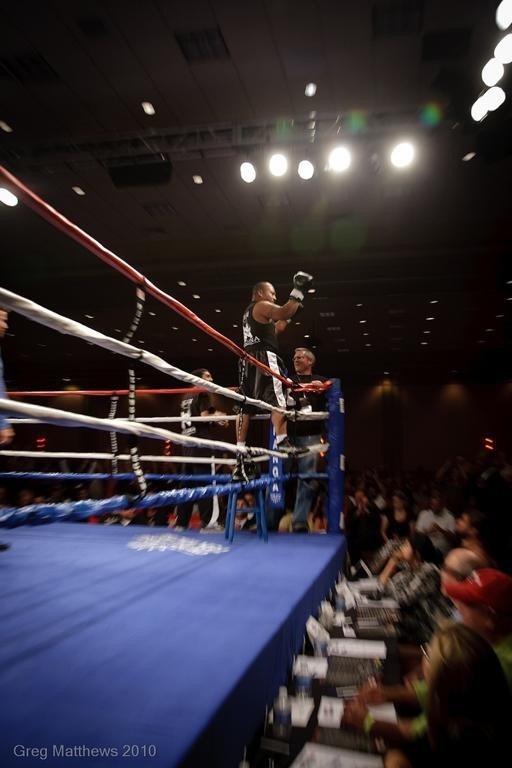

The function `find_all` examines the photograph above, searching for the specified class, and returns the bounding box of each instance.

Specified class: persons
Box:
[290,554,511,766]
[260,346,332,533]
[10,433,509,554]
[231,271,315,484]
[1,308,19,554]
[174,367,232,533]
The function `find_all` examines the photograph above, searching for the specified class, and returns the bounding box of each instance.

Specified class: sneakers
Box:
[293,521,309,534]
[174,523,226,534]
[236,449,252,463]
[272,436,310,454]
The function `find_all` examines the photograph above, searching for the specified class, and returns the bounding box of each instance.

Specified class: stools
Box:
[225,480,268,544]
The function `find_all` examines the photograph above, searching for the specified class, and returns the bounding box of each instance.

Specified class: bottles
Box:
[336,588,345,612]
[316,629,328,659]
[273,686,292,740]
[295,656,311,701]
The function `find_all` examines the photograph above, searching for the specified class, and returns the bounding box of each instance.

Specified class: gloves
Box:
[289,270,314,302]
